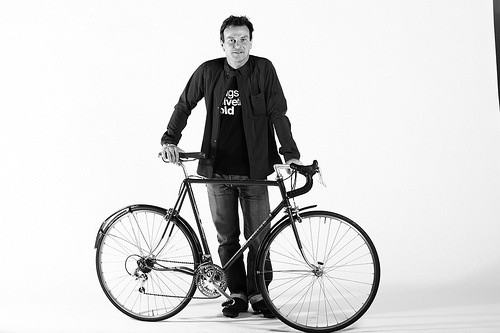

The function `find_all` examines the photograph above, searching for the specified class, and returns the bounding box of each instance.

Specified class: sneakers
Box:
[252,299,276,318]
[223,297,248,317]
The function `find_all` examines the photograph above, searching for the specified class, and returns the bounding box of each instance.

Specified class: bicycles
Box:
[94,152,381,332]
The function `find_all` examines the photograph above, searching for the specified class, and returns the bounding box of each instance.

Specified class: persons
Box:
[162,15,304,318]
